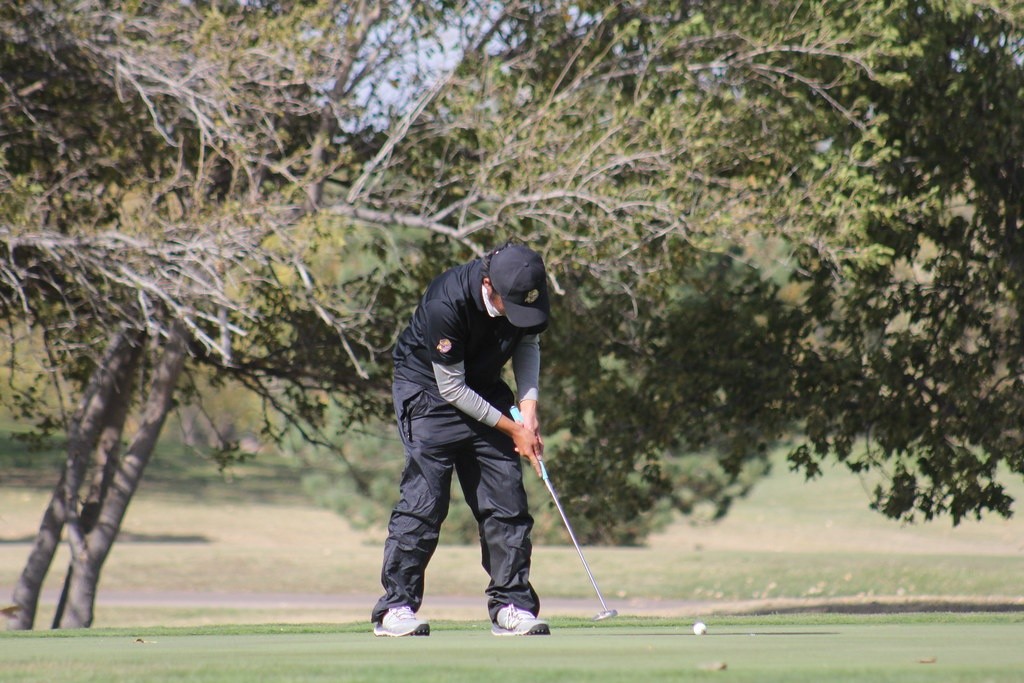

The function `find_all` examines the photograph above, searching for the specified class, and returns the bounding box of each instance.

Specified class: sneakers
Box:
[374,604,431,637]
[493,604,549,636]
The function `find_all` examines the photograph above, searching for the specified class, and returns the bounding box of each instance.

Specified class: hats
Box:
[489,245,550,328]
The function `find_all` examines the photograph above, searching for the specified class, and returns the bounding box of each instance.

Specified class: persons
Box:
[370,241,550,635]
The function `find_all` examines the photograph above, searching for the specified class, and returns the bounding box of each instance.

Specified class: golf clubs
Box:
[510,404,618,623]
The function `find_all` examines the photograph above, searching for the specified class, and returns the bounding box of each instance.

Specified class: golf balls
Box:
[693,623,707,635]
